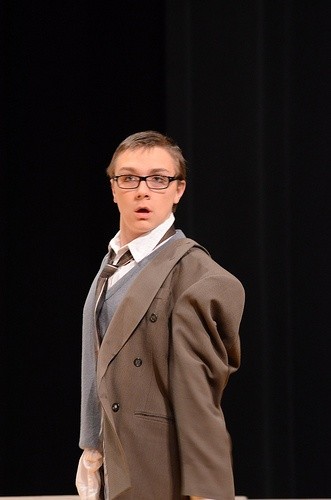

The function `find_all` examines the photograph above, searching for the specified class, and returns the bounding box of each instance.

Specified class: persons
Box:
[76,130,245,500]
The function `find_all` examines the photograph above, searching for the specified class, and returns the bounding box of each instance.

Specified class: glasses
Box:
[113,174,182,189]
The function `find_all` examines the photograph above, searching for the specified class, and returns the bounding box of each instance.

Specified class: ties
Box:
[93,226,176,371]
